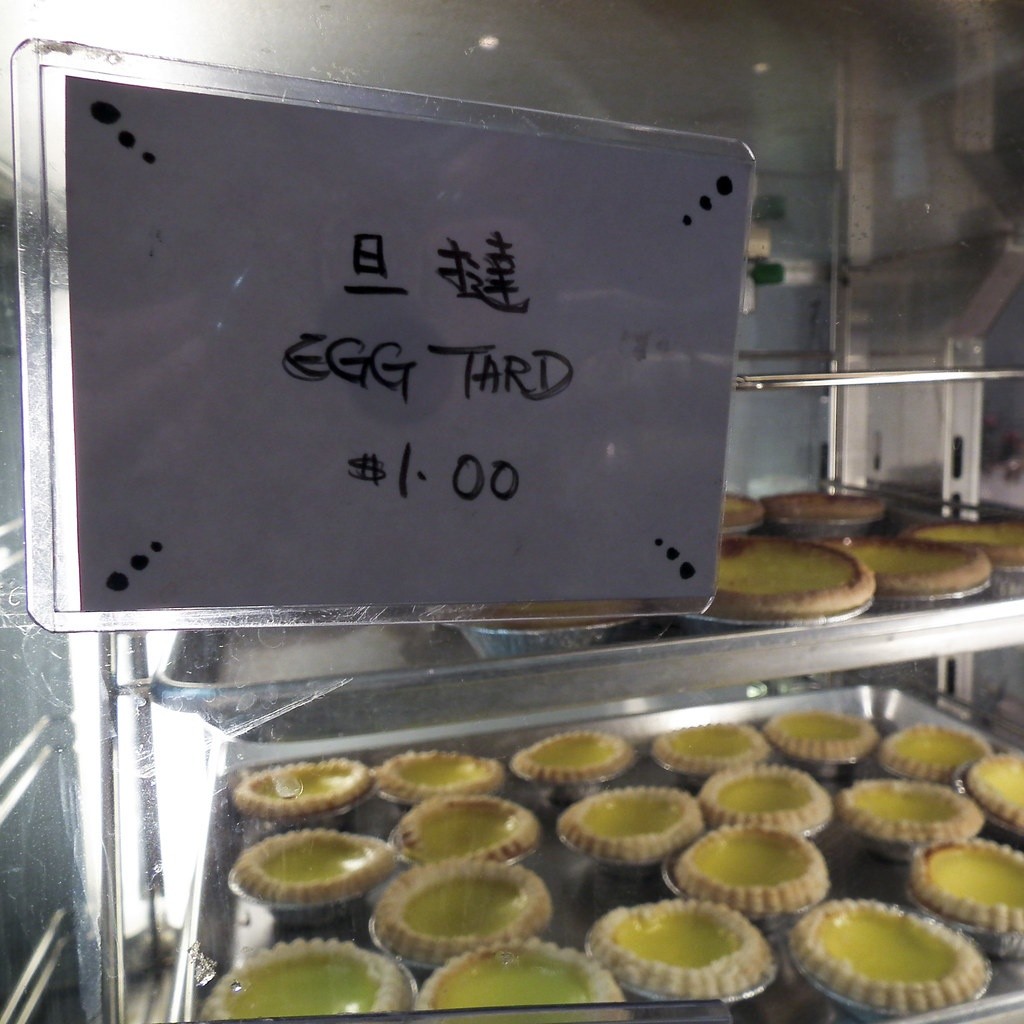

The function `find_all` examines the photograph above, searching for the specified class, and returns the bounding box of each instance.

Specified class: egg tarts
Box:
[454,493,1024,624]
[198,707,1024,1024]
